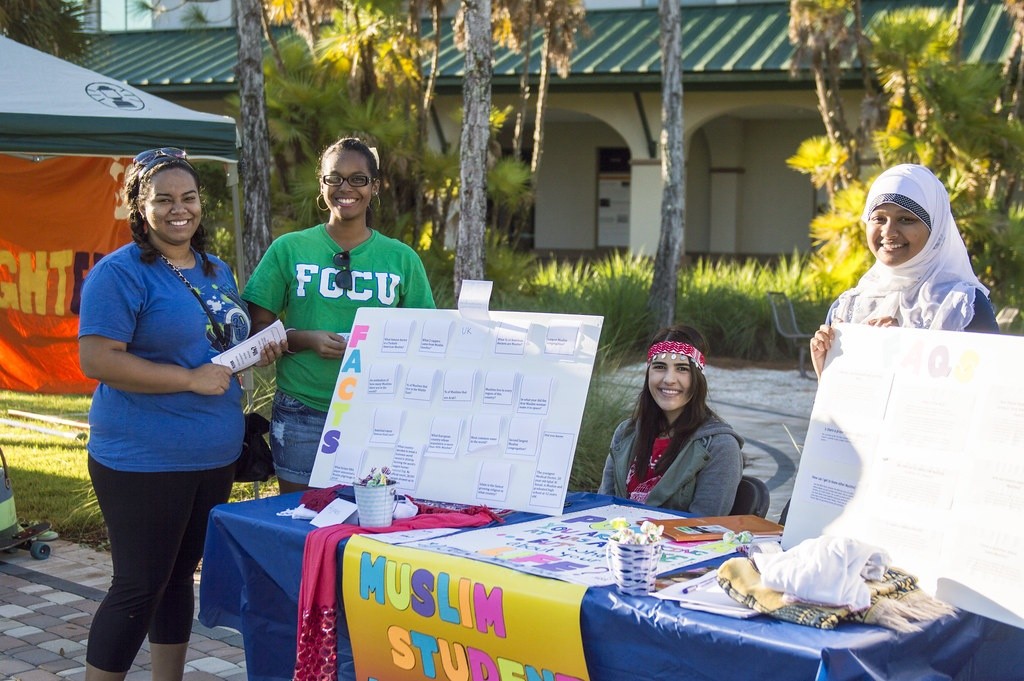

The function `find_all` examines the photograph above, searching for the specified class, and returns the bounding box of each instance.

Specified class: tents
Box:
[1,35,260,501]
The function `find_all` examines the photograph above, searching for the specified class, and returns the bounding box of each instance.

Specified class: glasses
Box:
[133,147,186,165]
[321,175,376,187]
[333,251,352,291]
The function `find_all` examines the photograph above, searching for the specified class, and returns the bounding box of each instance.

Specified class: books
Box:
[637,515,785,541]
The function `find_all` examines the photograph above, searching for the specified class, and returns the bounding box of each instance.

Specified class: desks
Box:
[200,492,1024,681]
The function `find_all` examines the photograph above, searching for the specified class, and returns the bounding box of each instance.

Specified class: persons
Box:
[597,325,745,517]
[241,137,435,495]
[77,147,288,681]
[809,164,1001,385]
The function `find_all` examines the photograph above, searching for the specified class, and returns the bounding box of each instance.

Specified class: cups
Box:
[607,537,662,597]
[352,479,398,528]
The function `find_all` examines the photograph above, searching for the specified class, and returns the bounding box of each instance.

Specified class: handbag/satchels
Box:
[234,413,275,483]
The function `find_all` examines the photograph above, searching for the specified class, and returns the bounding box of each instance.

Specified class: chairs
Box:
[729,475,770,518]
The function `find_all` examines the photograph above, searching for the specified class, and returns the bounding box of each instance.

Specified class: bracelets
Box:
[284,328,296,354]
